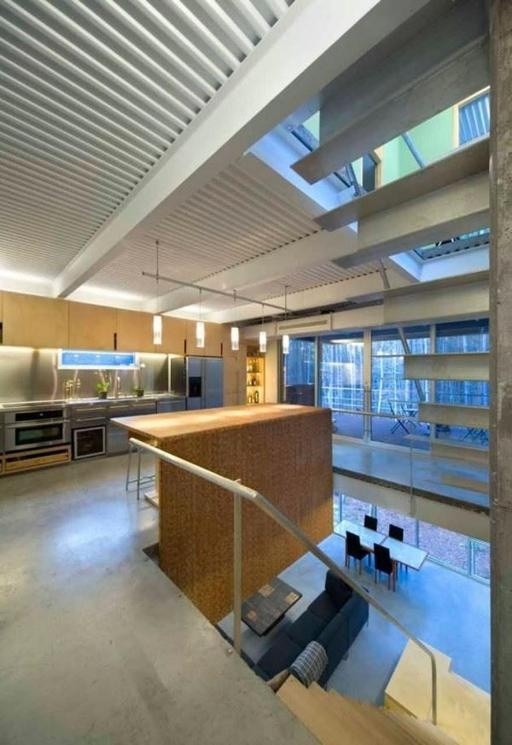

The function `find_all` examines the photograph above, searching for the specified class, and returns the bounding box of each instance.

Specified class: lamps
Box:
[141,239,294,356]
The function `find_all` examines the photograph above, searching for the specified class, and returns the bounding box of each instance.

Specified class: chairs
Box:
[387,398,420,434]
[345,514,404,591]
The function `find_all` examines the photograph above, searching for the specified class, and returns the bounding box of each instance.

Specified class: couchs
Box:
[257,568,369,688]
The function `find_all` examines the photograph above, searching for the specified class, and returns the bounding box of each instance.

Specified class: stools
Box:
[125,431,158,500]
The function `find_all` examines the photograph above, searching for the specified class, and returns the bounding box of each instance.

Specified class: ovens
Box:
[72,415,107,460]
[4,407,71,451]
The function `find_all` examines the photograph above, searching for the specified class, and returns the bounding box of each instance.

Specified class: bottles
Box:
[246,359,260,404]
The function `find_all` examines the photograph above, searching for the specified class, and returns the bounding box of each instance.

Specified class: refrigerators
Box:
[171,357,223,409]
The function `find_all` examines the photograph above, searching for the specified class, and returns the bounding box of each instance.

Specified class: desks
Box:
[331,520,429,579]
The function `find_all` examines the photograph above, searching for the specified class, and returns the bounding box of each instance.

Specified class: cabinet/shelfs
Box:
[1,290,68,351]
[186,319,227,358]
[68,302,155,354]
[107,400,157,454]
[155,314,187,355]
[157,397,186,414]
